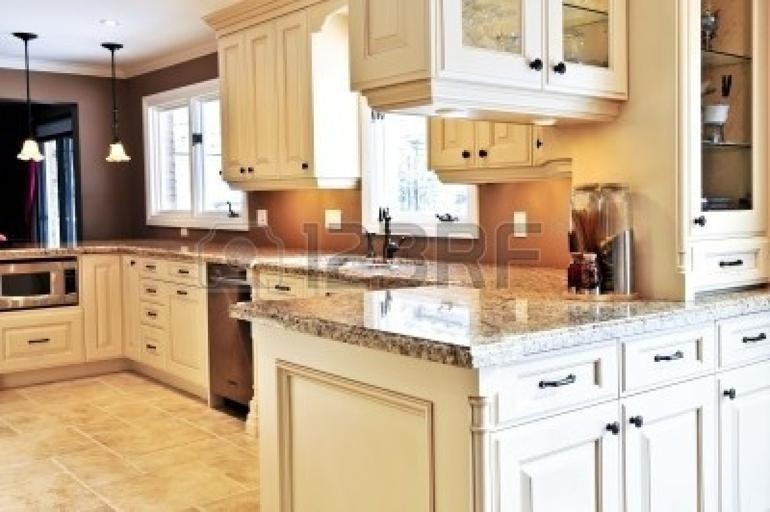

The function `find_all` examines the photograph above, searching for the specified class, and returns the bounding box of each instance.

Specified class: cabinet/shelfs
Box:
[0,0,770,512]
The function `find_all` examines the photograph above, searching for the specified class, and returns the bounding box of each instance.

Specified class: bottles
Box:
[567,253,600,296]
[599,182,636,297]
[569,182,599,252]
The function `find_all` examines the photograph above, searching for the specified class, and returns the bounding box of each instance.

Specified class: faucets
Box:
[378,208,406,257]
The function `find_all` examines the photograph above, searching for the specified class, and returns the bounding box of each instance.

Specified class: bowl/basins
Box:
[701,105,730,125]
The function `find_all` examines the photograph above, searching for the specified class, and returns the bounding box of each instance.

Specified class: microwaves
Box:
[1,258,80,312]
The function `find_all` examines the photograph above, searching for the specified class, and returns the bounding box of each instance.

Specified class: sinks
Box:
[298,259,426,270]
